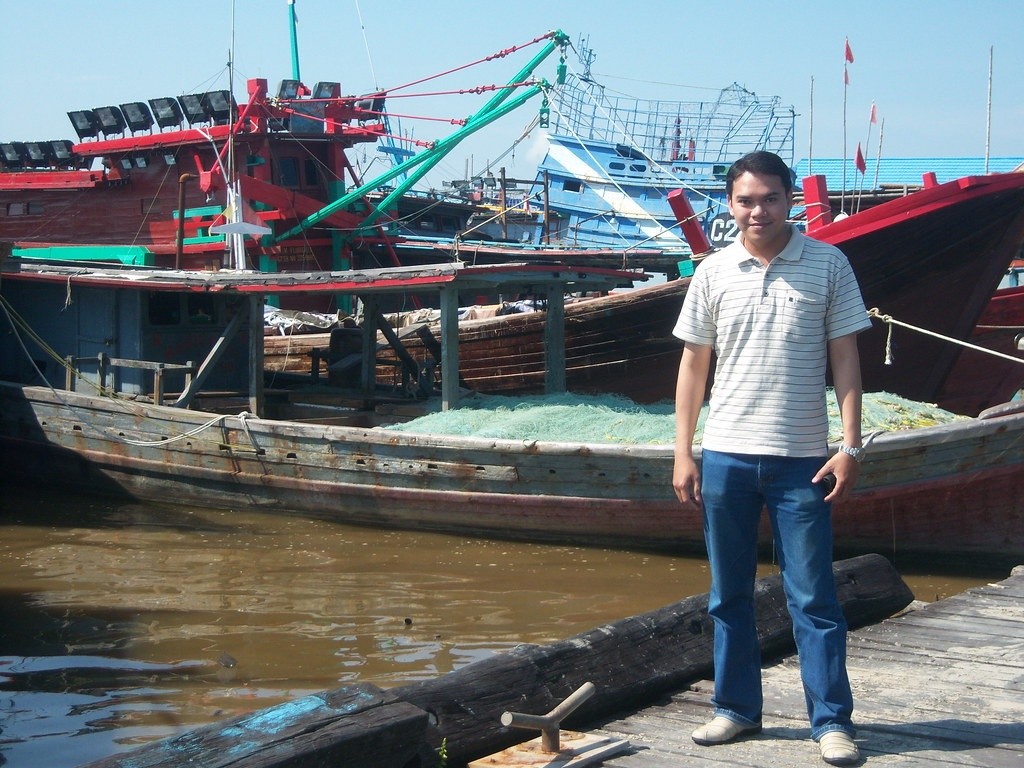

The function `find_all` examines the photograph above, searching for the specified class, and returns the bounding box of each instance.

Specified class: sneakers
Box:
[819,731,860,764]
[691,715,763,745]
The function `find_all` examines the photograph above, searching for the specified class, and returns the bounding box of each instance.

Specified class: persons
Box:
[671,151,874,763]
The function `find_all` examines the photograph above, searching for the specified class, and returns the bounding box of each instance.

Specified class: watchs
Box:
[839,446,865,463]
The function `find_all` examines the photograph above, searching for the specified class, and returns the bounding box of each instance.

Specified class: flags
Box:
[871,107,877,122]
[855,148,865,174]
[846,45,854,62]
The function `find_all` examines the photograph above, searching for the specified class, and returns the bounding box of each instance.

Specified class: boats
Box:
[1,0,1024,557]
